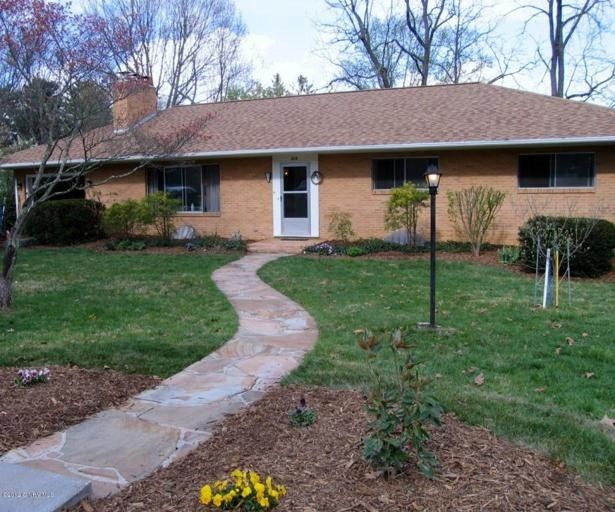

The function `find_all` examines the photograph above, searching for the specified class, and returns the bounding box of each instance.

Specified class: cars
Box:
[159,186,197,210]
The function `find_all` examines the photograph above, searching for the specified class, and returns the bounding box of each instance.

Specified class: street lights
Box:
[423,164,445,327]
